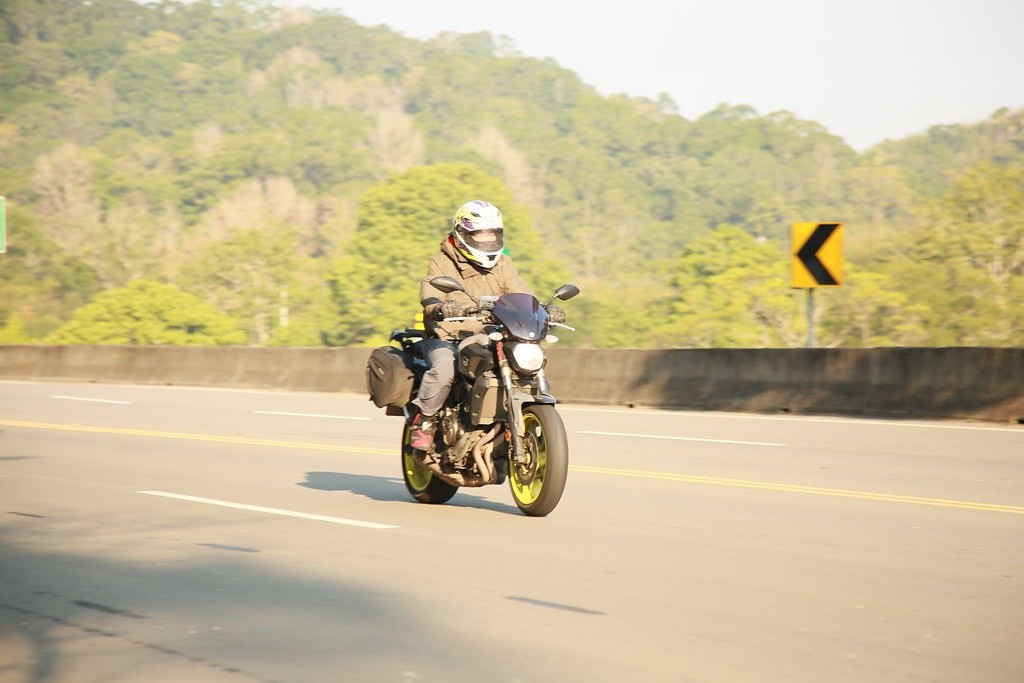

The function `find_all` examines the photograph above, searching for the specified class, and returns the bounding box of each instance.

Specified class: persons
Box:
[410,200,566,450]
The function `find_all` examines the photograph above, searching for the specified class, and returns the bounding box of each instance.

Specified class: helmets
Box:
[454,199,504,270]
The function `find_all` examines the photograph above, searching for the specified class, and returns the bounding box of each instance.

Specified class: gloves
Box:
[546,306,567,323]
[439,302,465,322]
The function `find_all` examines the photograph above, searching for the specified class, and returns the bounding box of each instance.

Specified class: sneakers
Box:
[409,414,437,451]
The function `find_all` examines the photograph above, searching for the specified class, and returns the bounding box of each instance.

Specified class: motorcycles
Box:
[389,278,582,518]
